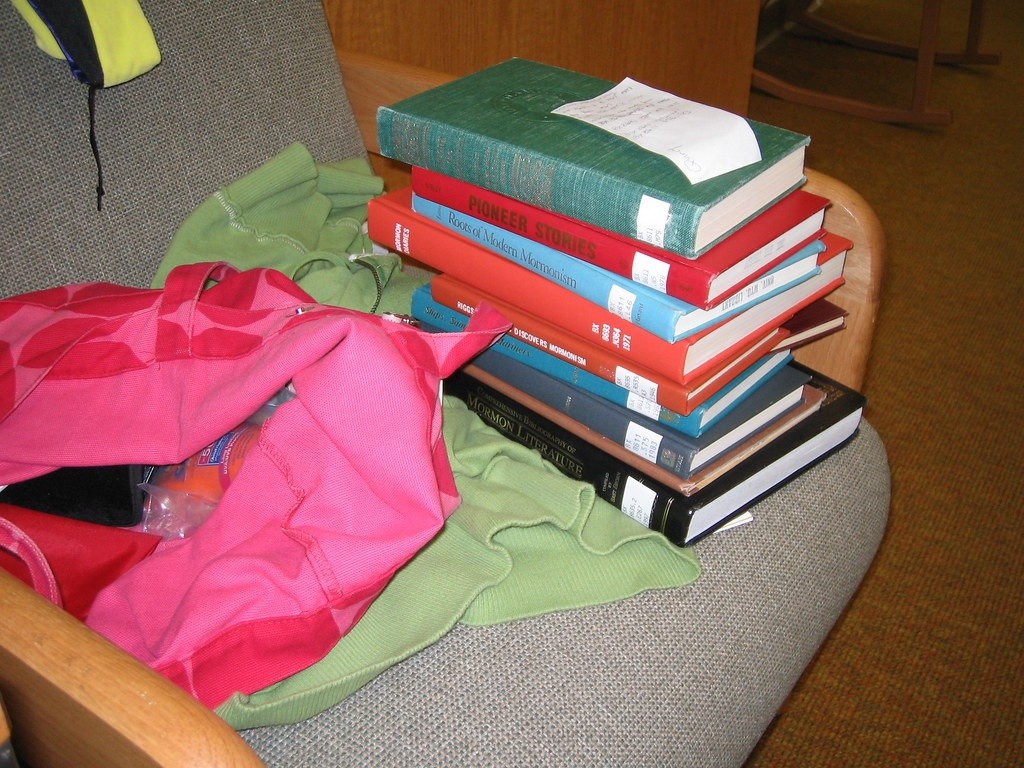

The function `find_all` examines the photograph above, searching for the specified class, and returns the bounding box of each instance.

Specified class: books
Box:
[368,57,868,549]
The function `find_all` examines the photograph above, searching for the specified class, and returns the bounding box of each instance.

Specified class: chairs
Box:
[0,0,892,768]
